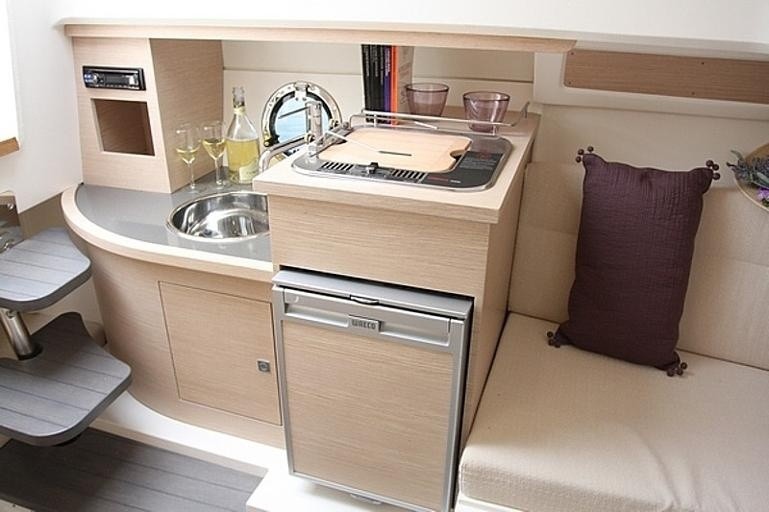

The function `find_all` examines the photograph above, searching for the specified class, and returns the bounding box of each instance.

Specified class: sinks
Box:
[166,189,270,242]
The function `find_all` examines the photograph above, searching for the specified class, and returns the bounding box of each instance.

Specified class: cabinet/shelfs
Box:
[65,28,227,197]
[0,223,134,449]
[250,103,543,511]
[79,230,289,452]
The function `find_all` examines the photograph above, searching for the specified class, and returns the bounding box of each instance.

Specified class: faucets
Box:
[258,100,324,174]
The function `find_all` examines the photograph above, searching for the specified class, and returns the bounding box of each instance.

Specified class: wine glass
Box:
[176,121,206,196]
[201,119,231,190]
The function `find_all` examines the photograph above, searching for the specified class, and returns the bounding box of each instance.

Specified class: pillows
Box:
[546,147,722,378]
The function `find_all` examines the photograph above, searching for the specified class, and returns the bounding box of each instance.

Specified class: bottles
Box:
[226,87,260,185]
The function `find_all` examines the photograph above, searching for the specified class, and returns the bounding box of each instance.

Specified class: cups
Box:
[404,82,450,123]
[462,91,510,134]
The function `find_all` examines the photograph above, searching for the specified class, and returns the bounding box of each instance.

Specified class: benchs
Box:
[451,103,769,512]
